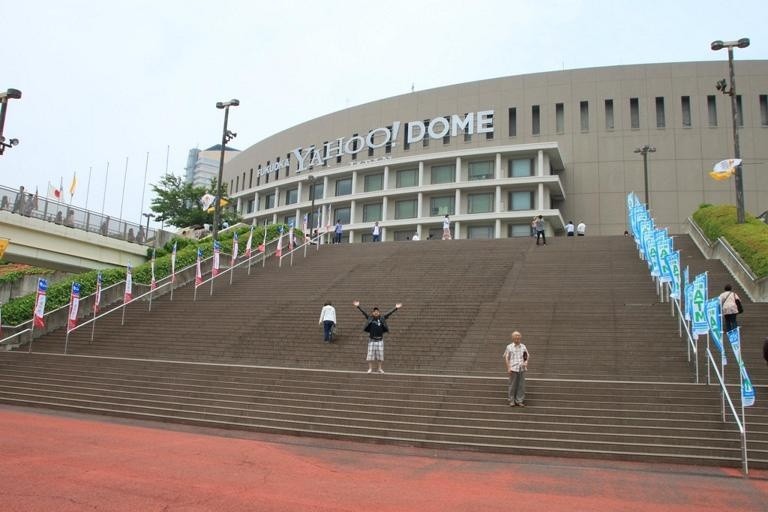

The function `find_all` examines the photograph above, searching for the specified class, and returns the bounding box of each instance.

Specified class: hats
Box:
[373,307,380,312]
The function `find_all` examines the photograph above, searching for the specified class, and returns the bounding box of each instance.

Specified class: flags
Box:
[70,175,78,193]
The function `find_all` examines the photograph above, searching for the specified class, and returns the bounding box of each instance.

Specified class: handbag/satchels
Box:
[331,325,338,337]
[523,352,527,360]
[735,298,744,315]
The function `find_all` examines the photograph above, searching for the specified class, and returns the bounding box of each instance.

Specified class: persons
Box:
[503,330,530,408]
[373,221,380,241]
[412,232,419,240]
[623,228,628,236]
[319,299,337,342]
[442,214,453,240]
[1,186,146,244]
[720,283,741,333]
[353,300,405,373]
[406,236,410,240]
[763,337,768,361]
[535,215,547,246]
[565,220,574,236]
[576,219,587,237]
[335,218,343,244]
[531,216,537,235]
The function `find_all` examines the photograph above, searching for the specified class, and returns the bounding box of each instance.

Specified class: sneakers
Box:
[510,402,525,408]
[367,368,384,373]
